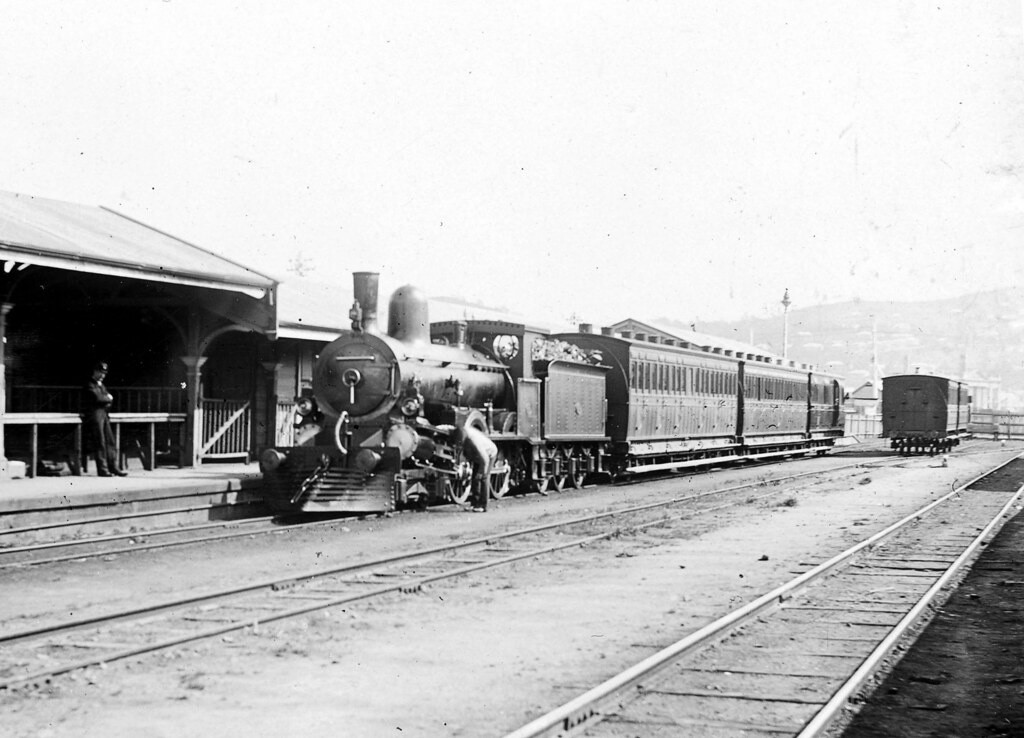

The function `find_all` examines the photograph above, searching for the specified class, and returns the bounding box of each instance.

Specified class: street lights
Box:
[781,287,793,356]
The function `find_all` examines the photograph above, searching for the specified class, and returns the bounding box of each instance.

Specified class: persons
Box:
[451,425,498,512]
[82,363,128,477]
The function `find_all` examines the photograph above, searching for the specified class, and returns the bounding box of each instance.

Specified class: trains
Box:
[877,374,973,457]
[259,270,853,514]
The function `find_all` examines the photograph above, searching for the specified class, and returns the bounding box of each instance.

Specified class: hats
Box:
[94,363,108,373]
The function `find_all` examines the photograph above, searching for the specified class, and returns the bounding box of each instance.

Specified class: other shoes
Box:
[109,469,128,476]
[98,472,114,477]
